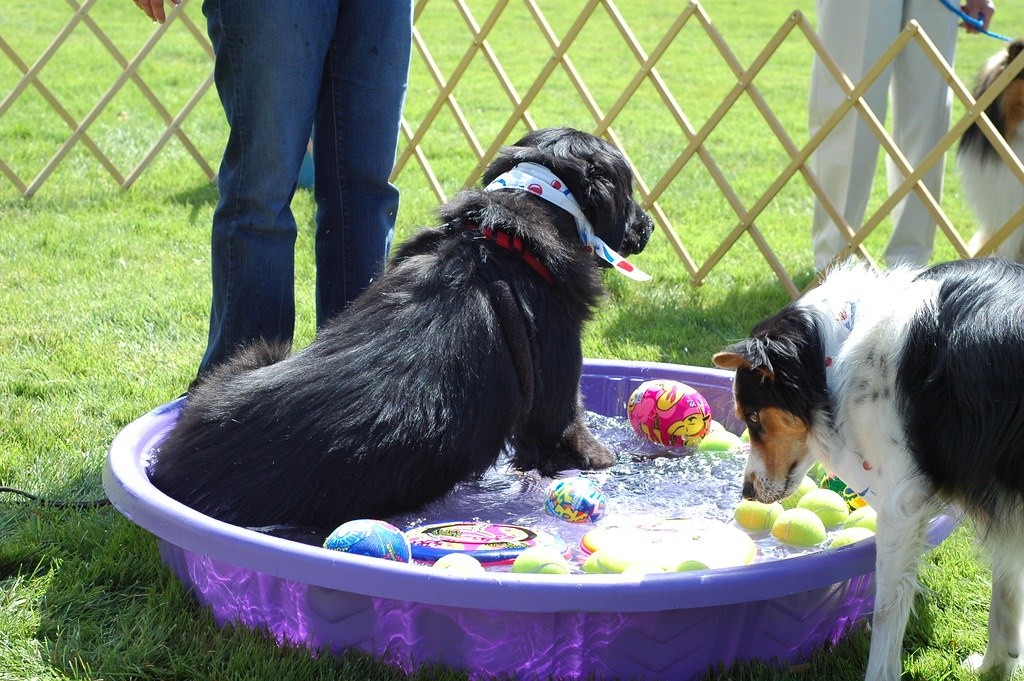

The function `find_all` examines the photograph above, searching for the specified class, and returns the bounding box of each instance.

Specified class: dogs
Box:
[714,256,1024,681]
[961,37,1024,256]
[154,125,657,543]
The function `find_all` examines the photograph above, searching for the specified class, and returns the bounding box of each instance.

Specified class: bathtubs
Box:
[106,356,969,681]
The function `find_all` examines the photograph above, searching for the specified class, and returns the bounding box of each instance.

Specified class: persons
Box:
[131,0,415,380]
[809,0,995,273]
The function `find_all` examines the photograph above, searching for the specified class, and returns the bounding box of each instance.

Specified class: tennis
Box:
[829,527,876,550]
[706,419,726,434]
[740,427,751,444]
[735,500,784,531]
[677,560,709,570]
[511,546,570,574]
[779,474,818,508]
[795,489,850,529]
[844,505,877,529]
[771,508,826,546]
[698,431,740,451]
[432,553,485,572]
[582,549,626,573]
